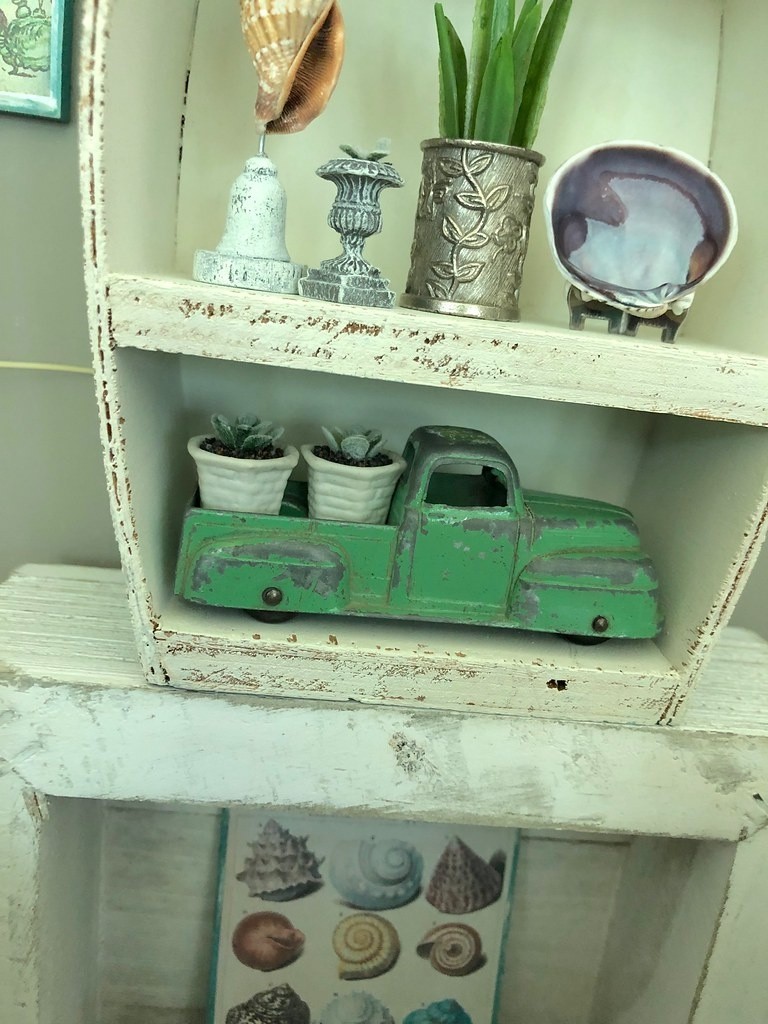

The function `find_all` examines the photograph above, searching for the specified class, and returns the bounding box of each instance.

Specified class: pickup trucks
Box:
[175,424,665,646]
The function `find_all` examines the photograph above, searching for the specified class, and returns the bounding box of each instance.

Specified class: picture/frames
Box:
[0,0,72,125]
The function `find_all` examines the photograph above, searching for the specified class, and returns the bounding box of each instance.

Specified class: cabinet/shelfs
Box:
[69,0,768,730]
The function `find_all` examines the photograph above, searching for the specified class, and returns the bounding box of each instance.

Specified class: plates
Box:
[542,141,740,321]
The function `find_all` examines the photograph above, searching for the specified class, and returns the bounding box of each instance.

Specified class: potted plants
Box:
[401,0,573,325]
[298,421,406,525]
[186,407,303,517]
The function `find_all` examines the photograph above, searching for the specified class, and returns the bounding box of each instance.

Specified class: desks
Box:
[0,563,768,1024]
